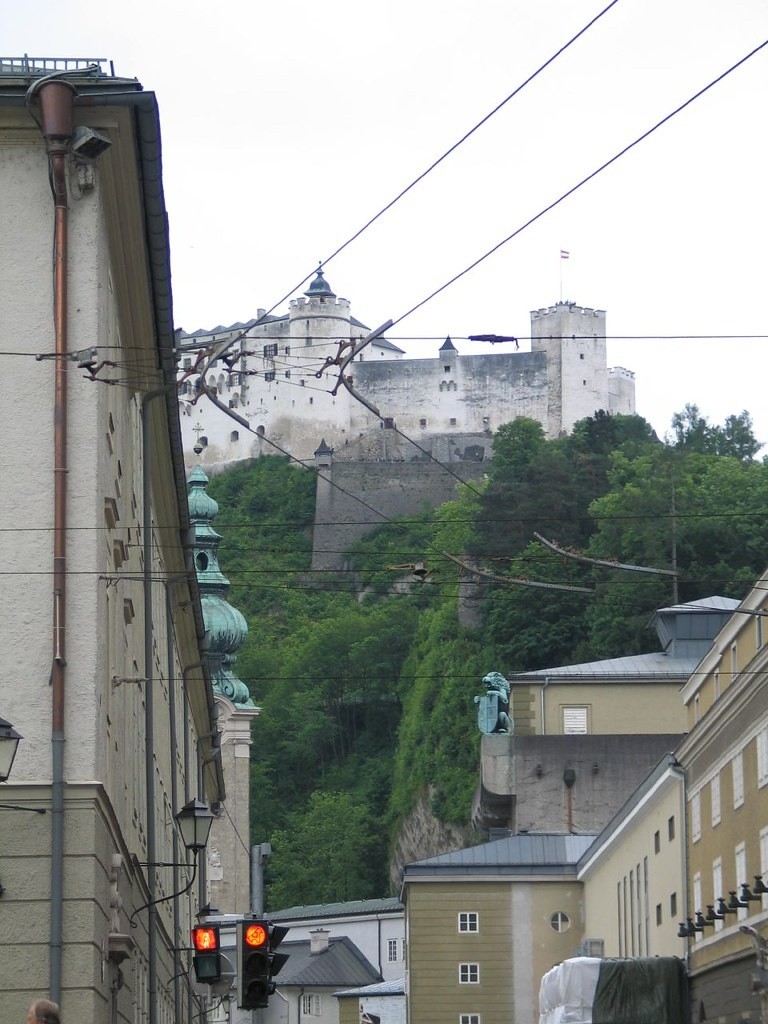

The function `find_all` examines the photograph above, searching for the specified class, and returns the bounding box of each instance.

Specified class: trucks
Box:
[539,956,691,1024]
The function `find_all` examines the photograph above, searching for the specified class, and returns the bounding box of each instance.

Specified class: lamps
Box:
[0,716,46,815]
[128,798,216,928]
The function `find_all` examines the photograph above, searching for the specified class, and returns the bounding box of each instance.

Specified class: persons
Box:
[474,672,511,733]
[27,999,60,1024]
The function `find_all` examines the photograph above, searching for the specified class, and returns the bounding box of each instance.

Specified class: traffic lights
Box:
[241,918,268,1009]
[192,924,221,982]
[268,922,290,995]
